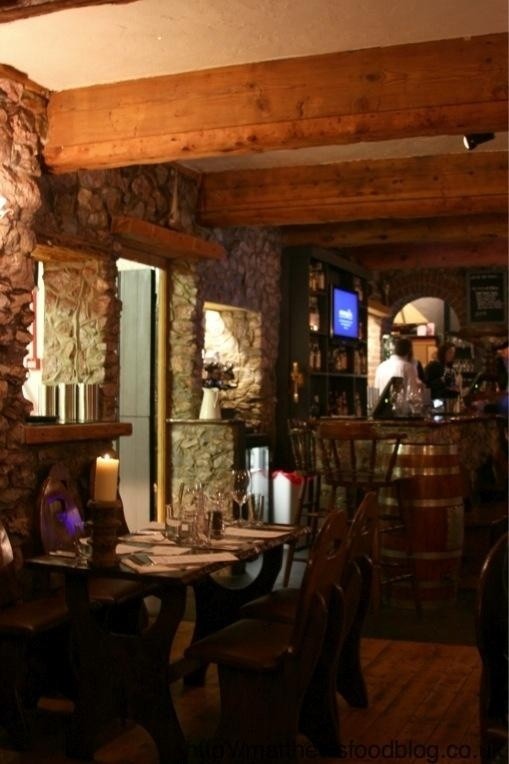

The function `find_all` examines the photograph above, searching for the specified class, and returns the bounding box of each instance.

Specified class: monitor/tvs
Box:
[327,279,361,341]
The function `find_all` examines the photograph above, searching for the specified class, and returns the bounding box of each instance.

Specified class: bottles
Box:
[209,511,225,539]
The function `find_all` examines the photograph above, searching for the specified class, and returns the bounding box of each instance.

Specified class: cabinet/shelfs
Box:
[277,242,369,421]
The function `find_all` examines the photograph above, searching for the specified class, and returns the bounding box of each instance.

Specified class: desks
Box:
[27,517,314,764]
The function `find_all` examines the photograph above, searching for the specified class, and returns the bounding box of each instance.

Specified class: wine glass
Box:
[226,468,250,526]
[181,481,207,546]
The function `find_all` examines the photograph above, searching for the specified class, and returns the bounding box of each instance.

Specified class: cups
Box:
[38,382,100,425]
[74,523,94,567]
[394,398,423,418]
[165,505,186,541]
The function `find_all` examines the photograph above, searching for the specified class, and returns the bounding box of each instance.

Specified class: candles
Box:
[94,453,120,502]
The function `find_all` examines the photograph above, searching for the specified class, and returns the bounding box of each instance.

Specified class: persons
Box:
[471,395,509,417]
[422,340,464,402]
[375,337,428,409]
[492,341,509,393]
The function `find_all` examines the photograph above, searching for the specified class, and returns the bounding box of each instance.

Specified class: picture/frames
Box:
[466,267,507,326]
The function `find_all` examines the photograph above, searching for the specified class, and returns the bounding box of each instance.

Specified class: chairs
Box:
[184,491,378,764]
[0,477,140,752]
[286,416,424,618]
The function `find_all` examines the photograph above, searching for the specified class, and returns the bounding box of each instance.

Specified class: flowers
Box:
[201,361,235,390]
